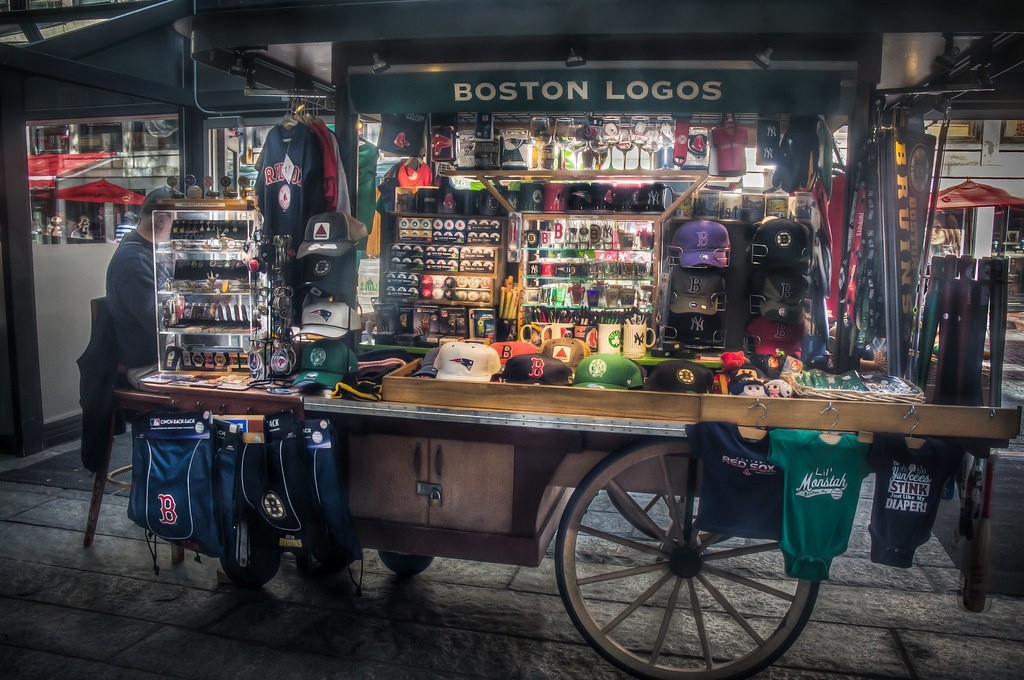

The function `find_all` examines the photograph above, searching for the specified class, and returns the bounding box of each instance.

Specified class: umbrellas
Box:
[31,178,146,240]
[936,177,1024,256]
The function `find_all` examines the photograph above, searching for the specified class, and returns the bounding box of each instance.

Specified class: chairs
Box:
[84,297,202,565]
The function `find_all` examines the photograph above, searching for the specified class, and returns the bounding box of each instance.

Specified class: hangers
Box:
[359,136,368,146]
[278,93,321,128]
[814,408,843,444]
[898,410,927,448]
[405,154,423,168]
[737,402,770,441]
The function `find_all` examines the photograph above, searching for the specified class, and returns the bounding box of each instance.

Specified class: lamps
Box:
[565,47,587,69]
[372,51,389,73]
[755,47,774,69]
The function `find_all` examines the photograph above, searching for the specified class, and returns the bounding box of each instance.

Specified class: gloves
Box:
[768,114,822,191]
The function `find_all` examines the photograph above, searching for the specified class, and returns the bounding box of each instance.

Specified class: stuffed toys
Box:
[712,351,792,398]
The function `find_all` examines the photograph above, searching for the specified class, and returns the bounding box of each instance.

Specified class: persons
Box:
[116,211,139,243]
[105,186,189,393]
[927,214,961,265]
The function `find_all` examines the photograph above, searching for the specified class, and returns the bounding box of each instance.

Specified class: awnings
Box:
[28,152,117,189]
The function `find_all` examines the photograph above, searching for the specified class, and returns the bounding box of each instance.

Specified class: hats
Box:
[658,223,807,358]
[289,208,367,391]
[410,340,773,401]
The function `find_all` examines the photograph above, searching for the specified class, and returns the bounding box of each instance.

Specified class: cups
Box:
[672,190,815,222]
[523,220,653,250]
[528,257,648,278]
[680,126,708,169]
[623,323,656,357]
[456,127,529,170]
[394,180,672,216]
[523,282,654,309]
[597,324,621,354]
[541,323,574,342]
[562,325,598,352]
[496,318,517,341]
[520,320,552,349]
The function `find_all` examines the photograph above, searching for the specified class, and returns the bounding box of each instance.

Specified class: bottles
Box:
[366,316,373,343]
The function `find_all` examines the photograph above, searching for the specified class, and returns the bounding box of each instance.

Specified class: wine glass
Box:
[530,117,677,171]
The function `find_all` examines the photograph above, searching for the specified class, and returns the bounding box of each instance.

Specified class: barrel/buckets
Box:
[358,258,379,304]
